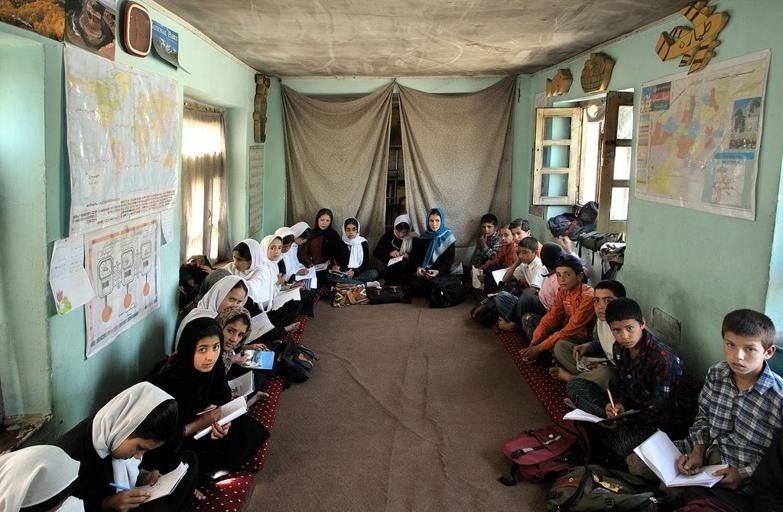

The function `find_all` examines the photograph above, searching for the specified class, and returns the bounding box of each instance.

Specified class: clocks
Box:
[120,2,153,57]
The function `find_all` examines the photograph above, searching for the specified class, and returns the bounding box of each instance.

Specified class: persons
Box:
[402,207,458,300]
[309,207,349,299]
[1,221,319,511]
[467,214,783,511]
[325,216,386,289]
[370,213,421,287]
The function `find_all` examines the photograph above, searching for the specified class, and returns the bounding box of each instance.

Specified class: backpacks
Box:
[544,463,669,512]
[599,242,625,280]
[497,417,589,486]
[470,284,521,327]
[657,484,752,512]
[548,212,576,238]
[566,201,599,241]
[575,230,623,266]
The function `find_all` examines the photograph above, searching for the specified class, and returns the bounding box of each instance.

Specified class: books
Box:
[328,269,348,278]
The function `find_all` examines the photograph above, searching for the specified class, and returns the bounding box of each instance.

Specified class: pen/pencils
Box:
[109,482,152,497]
[334,259,337,267]
[685,454,691,476]
[579,344,581,354]
[196,408,216,415]
[310,256,312,265]
[607,388,618,417]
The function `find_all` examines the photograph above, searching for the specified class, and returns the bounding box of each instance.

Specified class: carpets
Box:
[196,281,322,512]
[489,314,573,423]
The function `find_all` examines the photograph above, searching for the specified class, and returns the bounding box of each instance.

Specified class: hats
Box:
[555,254,588,283]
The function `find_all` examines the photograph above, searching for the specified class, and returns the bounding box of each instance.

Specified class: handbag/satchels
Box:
[329,282,368,308]
[276,335,320,389]
[366,282,411,304]
[427,274,465,308]
